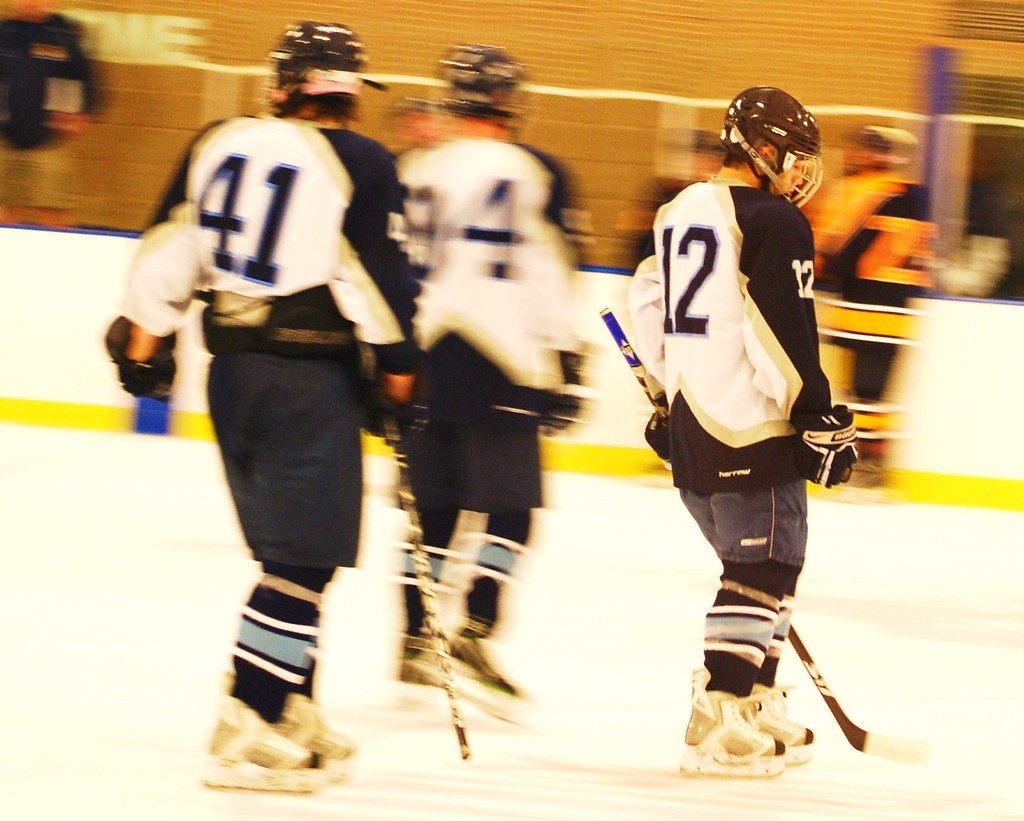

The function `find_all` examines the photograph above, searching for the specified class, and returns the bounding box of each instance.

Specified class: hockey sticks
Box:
[597,305,931,760]
[360,340,474,763]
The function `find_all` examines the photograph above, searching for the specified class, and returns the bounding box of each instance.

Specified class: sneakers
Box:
[197,669,350,793]
[677,667,789,778]
[396,632,475,717]
[437,631,531,731]
[277,691,360,772]
[744,682,813,768]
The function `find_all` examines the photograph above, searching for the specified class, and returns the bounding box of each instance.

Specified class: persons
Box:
[630,124,935,488]
[628,87,859,759]
[0,0,108,224]
[385,43,587,696]
[106,22,422,771]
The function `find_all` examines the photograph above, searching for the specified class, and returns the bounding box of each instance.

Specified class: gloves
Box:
[787,405,857,488]
[106,315,177,402]
[645,394,672,468]
[363,381,434,469]
[478,383,582,436]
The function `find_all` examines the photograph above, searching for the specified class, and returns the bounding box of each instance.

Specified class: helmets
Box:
[846,124,894,172]
[721,86,823,208]
[435,38,539,132]
[268,20,391,103]
